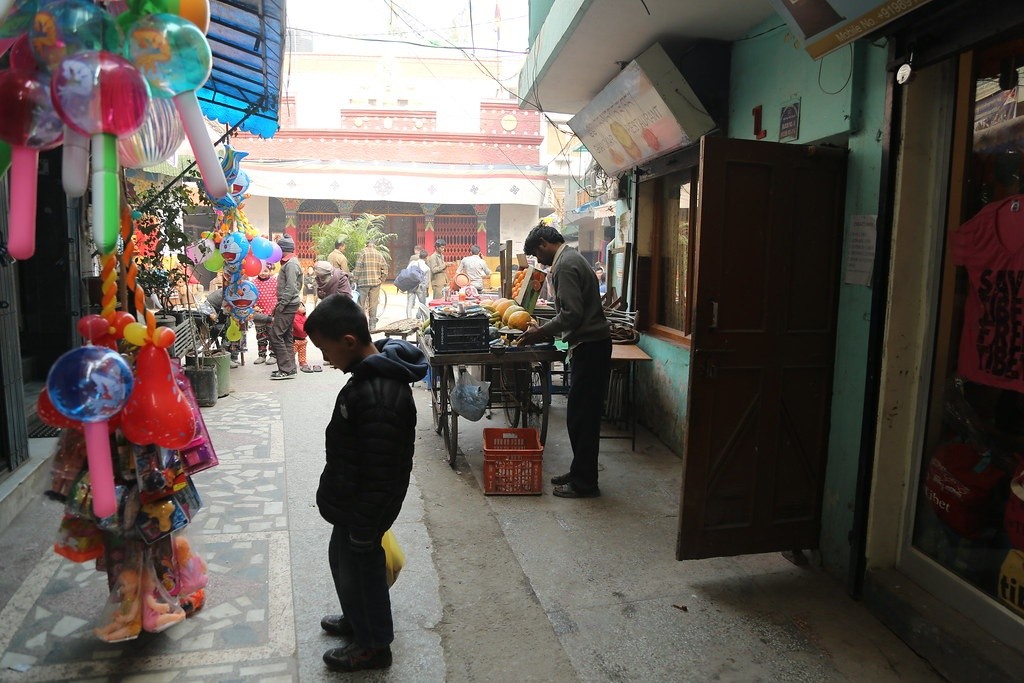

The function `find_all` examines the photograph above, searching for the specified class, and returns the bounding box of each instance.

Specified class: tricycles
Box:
[416,286,566,467]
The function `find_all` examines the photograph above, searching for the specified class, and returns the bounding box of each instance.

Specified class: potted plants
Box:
[186,296,233,399]
[136,164,218,408]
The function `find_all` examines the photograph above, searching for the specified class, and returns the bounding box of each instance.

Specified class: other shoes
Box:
[270,370,296,379]
[321,615,348,636]
[299,363,308,366]
[552,482,600,498]
[232,359,240,364]
[551,471,574,485]
[230,359,238,368]
[323,642,392,672]
[266,357,277,365]
[272,370,297,376]
[253,357,267,363]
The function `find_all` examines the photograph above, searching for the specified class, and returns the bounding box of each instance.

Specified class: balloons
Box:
[212,232,282,342]
[1,1,229,518]
[200,144,250,210]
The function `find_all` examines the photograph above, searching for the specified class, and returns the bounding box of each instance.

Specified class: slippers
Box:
[300,365,313,373]
[313,365,322,372]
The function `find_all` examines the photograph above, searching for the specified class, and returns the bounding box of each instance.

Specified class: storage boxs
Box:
[483,426,545,497]
[430,304,489,353]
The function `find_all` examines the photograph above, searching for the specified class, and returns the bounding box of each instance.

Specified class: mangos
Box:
[512,268,528,300]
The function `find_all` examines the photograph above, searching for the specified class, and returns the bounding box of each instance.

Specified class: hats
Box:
[313,260,333,276]
[277,233,294,252]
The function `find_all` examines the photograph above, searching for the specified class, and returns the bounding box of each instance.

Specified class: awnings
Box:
[138,1,286,214]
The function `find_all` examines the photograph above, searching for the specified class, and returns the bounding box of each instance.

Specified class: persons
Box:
[456,244,490,293]
[253,261,278,365]
[313,260,354,367]
[206,287,274,367]
[516,220,613,501]
[326,239,388,333]
[406,239,454,320]
[595,268,607,294]
[302,295,432,670]
[265,234,314,380]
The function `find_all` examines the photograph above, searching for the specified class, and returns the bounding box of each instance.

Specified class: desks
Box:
[599,343,654,452]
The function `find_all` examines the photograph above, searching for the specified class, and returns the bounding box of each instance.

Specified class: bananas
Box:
[482,305,501,328]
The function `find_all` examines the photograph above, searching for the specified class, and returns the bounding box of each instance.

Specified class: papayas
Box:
[480,297,532,331]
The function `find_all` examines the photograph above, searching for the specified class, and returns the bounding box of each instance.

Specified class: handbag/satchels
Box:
[381,528,404,589]
[450,364,491,421]
[393,262,424,293]
